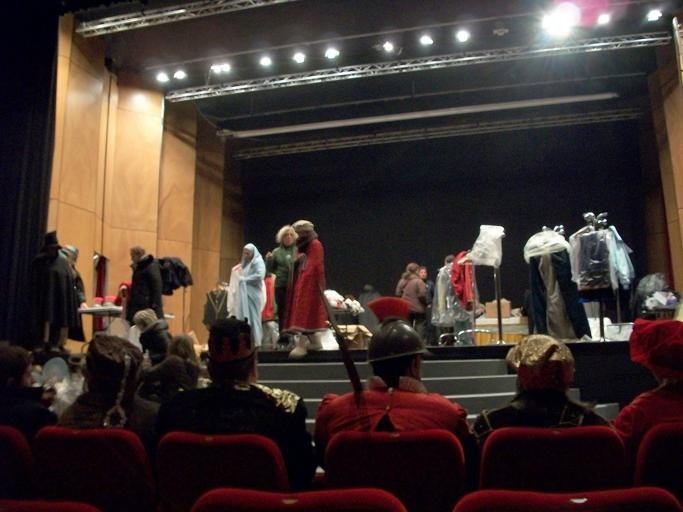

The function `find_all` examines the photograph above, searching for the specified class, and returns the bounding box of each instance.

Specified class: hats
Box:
[84,336,143,427]
[209,317,258,365]
[63,244,76,262]
[293,222,314,232]
[629,318,682,383]
[518,333,574,394]
[40,232,63,251]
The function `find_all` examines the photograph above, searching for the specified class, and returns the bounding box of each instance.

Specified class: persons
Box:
[59,244,88,343]
[466,335,611,450]
[165,334,197,364]
[264,225,301,345]
[445,255,454,268]
[125,246,164,324]
[227,244,264,344]
[116,279,130,306]
[418,265,435,345]
[148,357,192,402]
[31,231,75,345]
[57,336,160,440]
[610,320,681,448]
[285,220,327,357]
[1,343,56,442]
[159,318,319,488]
[134,309,157,332]
[396,262,427,306]
[313,320,464,453]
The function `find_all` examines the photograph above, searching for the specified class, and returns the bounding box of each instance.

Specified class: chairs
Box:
[187,488,406,512]
[0,498,98,512]
[151,433,287,512]
[32,427,157,512]
[322,430,465,512]
[636,421,682,504]
[452,488,681,512]
[0,424,48,501]
[478,425,631,497]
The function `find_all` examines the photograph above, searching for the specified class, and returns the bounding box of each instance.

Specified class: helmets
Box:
[366,318,427,362]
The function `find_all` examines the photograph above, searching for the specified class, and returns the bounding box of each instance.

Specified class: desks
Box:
[77,307,175,326]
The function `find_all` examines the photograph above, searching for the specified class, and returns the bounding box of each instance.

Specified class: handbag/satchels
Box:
[399,276,420,300]
[605,322,634,341]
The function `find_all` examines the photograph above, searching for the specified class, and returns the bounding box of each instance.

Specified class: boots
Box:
[306,334,323,350]
[288,335,309,360]
